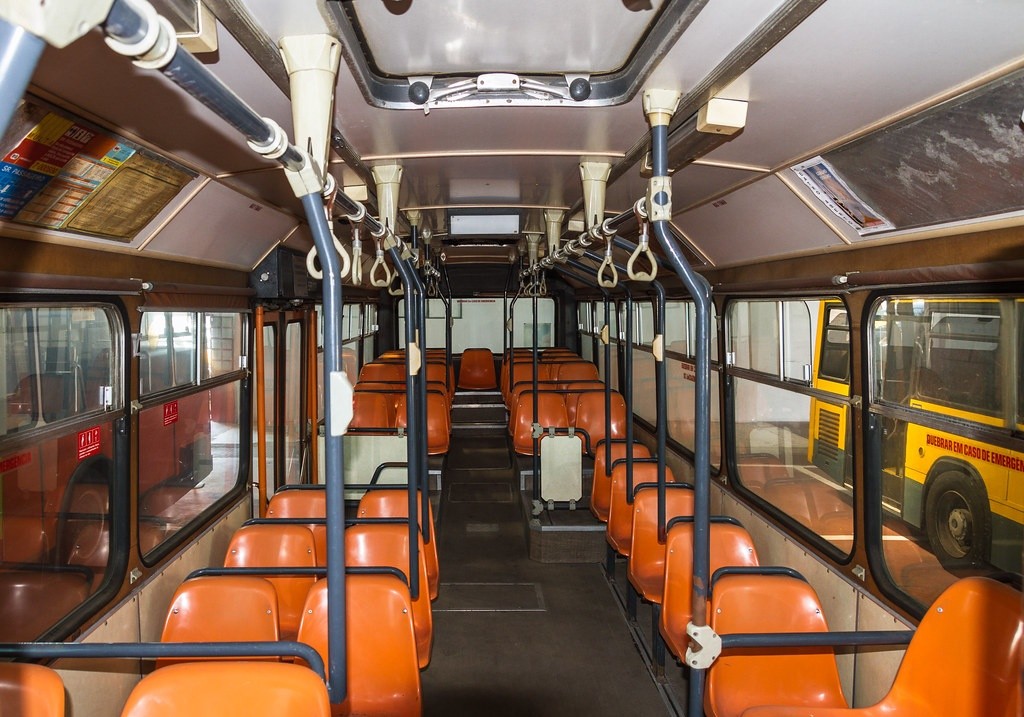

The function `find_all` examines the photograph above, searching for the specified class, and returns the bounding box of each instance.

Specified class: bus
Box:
[0,308,214,575]
[804,289,1024,577]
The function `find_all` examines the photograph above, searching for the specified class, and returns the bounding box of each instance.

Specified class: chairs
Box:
[0,662,65,717]
[590,442,651,523]
[345,524,434,669]
[265,490,327,567]
[356,489,440,601]
[501,338,640,457]
[8,348,109,413]
[628,489,695,684]
[458,348,498,390]
[118,661,332,717]
[223,524,318,661]
[347,347,455,457]
[154,576,281,670]
[704,574,849,717]
[658,523,761,666]
[741,576,1024,717]
[606,462,676,584]
[294,574,422,717]
